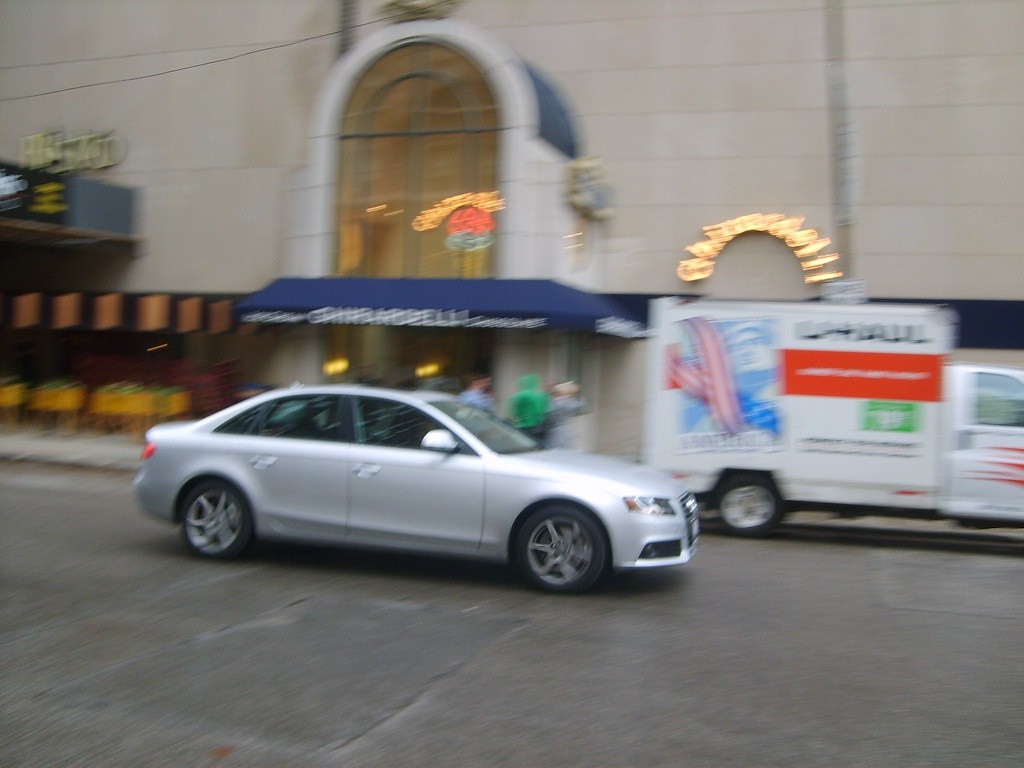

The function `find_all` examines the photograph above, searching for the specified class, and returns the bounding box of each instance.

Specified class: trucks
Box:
[642,294,1024,535]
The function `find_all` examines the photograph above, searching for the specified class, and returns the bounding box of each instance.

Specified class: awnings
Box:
[235,277,645,342]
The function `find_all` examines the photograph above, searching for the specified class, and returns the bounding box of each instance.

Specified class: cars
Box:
[132,381,699,592]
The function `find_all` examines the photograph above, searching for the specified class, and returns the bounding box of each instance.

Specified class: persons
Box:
[461,372,495,412]
[550,381,585,410]
[506,372,550,438]
